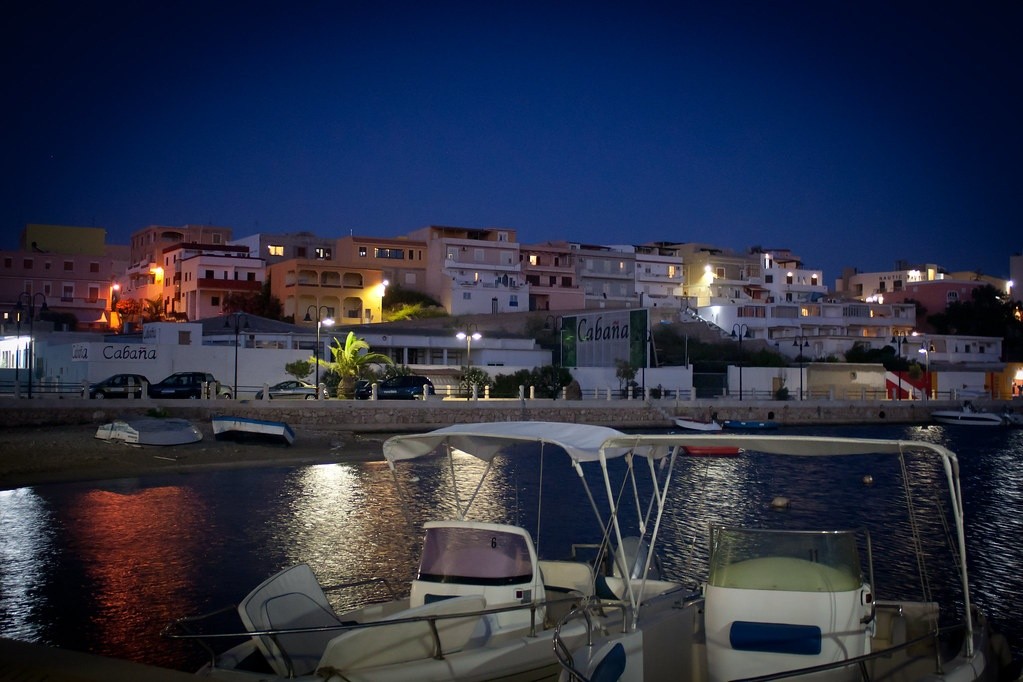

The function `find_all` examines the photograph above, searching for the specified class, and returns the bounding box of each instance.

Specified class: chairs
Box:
[175,378,184,383]
[119,378,126,385]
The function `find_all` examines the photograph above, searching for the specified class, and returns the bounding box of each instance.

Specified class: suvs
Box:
[376,375,436,400]
[149,371,221,400]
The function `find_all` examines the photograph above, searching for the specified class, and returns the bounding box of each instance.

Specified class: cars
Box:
[255,379,329,401]
[219,385,233,400]
[81,374,151,400]
[355,379,383,399]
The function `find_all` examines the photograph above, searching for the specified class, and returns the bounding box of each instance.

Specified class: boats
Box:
[721,419,783,432]
[209,415,295,445]
[930,410,1018,427]
[158,418,695,682]
[669,416,725,432]
[95,418,204,446]
[551,432,1011,682]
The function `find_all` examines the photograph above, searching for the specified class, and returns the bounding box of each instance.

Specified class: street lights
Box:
[222,314,252,400]
[540,315,568,367]
[890,330,908,402]
[792,335,810,401]
[13,292,50,399]
[455,321,483,401]
[919,343,936,401]
[631,329,651,400]
[729,323,752,401]
[302,304,336,399]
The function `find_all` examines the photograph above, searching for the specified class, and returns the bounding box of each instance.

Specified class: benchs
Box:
[539,559,619,599]
[556,608,626,682]
[238,563,486,676]
